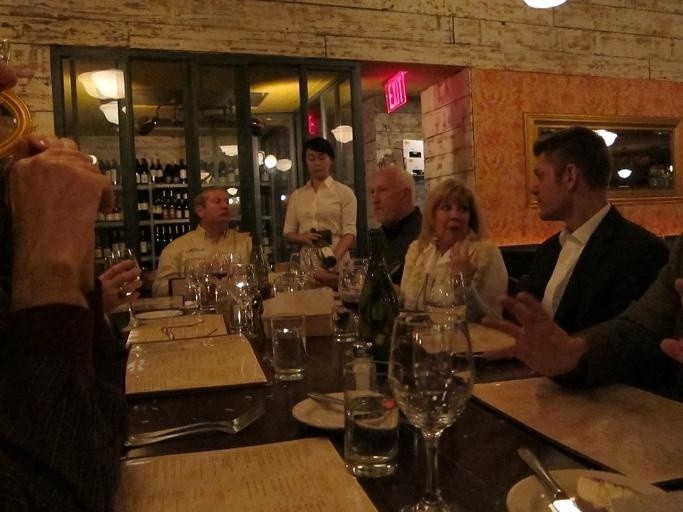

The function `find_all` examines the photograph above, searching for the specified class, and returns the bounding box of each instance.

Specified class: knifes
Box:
[515,445,585,512]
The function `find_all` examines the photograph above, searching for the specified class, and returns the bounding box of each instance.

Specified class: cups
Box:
[337,359,407,479]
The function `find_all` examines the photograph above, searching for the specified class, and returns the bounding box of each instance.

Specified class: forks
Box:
[123,405,266,447]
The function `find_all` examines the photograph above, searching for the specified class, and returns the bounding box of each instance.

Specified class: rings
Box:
[119,286,127,297]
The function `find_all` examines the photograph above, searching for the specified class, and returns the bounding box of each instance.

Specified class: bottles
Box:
[262,224,270,248]
[353,227,413,380]
[91,153,191,277]
[199,157,270,185]
[309,227,337,270]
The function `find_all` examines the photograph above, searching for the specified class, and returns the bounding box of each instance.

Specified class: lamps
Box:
[78,72,105,99]
[264,154,277,169]
[591,129,618,147]
[220,145,239,157]
[617,169,632,178]
[100,101,119,125]
[91,68,124,99]
[330,125,353,144]
[276,158,292,172]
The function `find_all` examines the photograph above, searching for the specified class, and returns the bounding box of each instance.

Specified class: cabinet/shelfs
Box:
[93,181,278,272]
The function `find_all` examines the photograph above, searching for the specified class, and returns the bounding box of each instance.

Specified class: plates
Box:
[134,309,185,321]
[290,393,401,430]
[407,317,520,356]
[504,468,674,511]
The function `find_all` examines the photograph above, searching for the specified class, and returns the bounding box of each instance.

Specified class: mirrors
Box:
[523,112,683,208]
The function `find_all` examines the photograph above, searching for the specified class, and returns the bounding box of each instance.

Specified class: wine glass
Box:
[181,242,474,391]
[102,246,147,333]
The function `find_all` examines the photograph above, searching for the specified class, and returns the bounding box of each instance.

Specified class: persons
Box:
[0,38,132,512]
[400,179,508,325]
[283,137,357,268]
[367,166,423,295]
[530,127,669,391]
[481,232,683,390]
[99,258,143,316]
[151,185,253,297]
